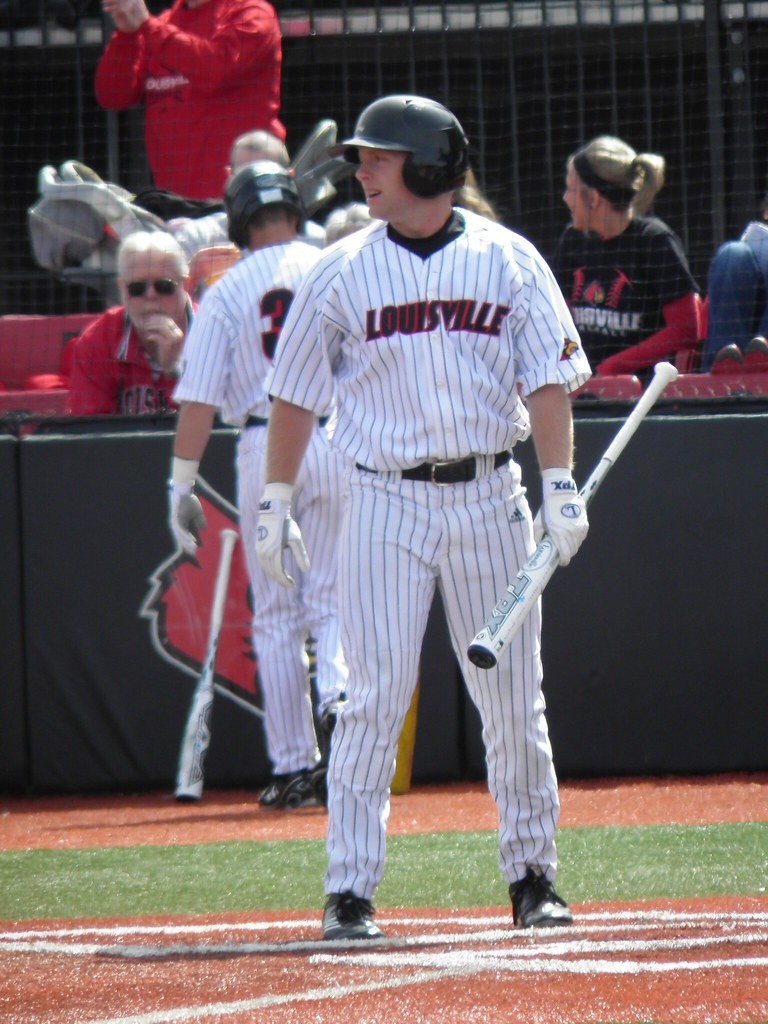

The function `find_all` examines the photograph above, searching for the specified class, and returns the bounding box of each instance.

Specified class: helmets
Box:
[327,94,472,200]
[223,160,305,247]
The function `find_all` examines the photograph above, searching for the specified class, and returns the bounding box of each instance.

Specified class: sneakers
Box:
[257,768,318,809]
[508,869,573,928]
[319,889,383,939]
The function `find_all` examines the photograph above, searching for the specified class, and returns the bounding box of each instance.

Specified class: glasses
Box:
[121,274,186,299]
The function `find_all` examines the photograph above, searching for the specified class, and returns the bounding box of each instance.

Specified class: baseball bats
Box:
[467,361,679,670]
[174,530,240,803]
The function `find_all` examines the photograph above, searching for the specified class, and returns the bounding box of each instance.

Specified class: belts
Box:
[244,415,331,431]
[355,450,513,487]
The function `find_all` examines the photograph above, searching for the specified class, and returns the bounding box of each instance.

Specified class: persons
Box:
[166,161,352,809]
[95,1,284,202]
[704,200,768,370]
[174,130,326,261]
[257,97,591,942]
[70,230,202,416]
[550,138,702,383]
[325,205,372,244]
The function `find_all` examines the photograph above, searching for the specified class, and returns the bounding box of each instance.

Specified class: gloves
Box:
[168,457,207,556]
[255,482,310,588]
[531,467,589,566]
